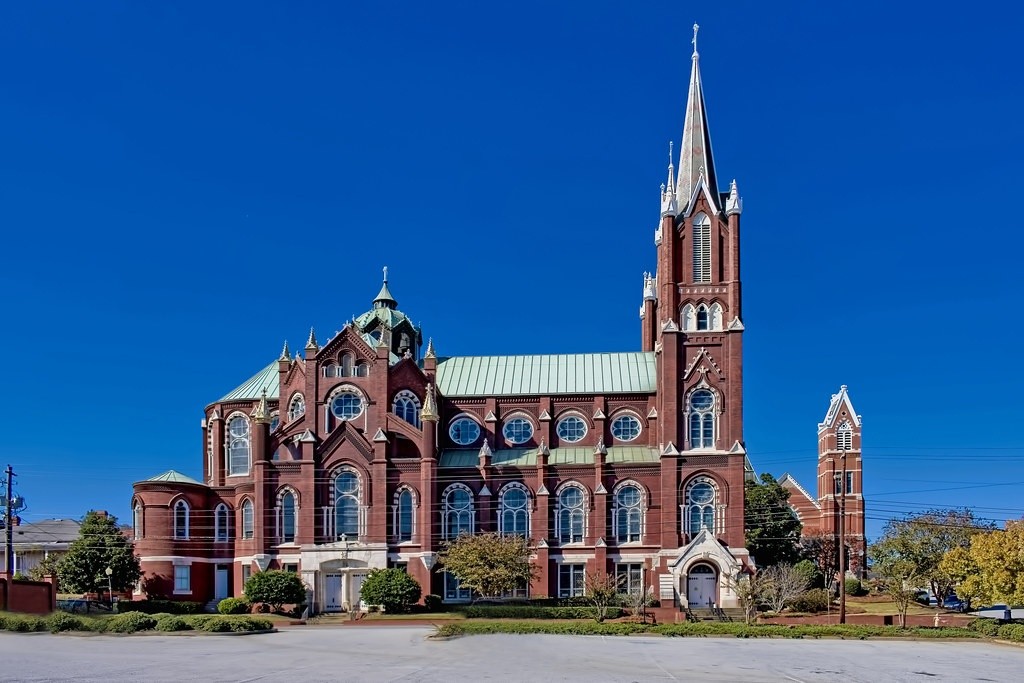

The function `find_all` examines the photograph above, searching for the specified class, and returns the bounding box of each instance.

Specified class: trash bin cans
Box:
[1004,610,1012,619]
[884,616,893,625]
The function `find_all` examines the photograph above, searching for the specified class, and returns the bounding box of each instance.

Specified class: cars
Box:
[917,591,972,612]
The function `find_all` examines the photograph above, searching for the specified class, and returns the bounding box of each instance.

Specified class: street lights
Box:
[105,566,113,602]
